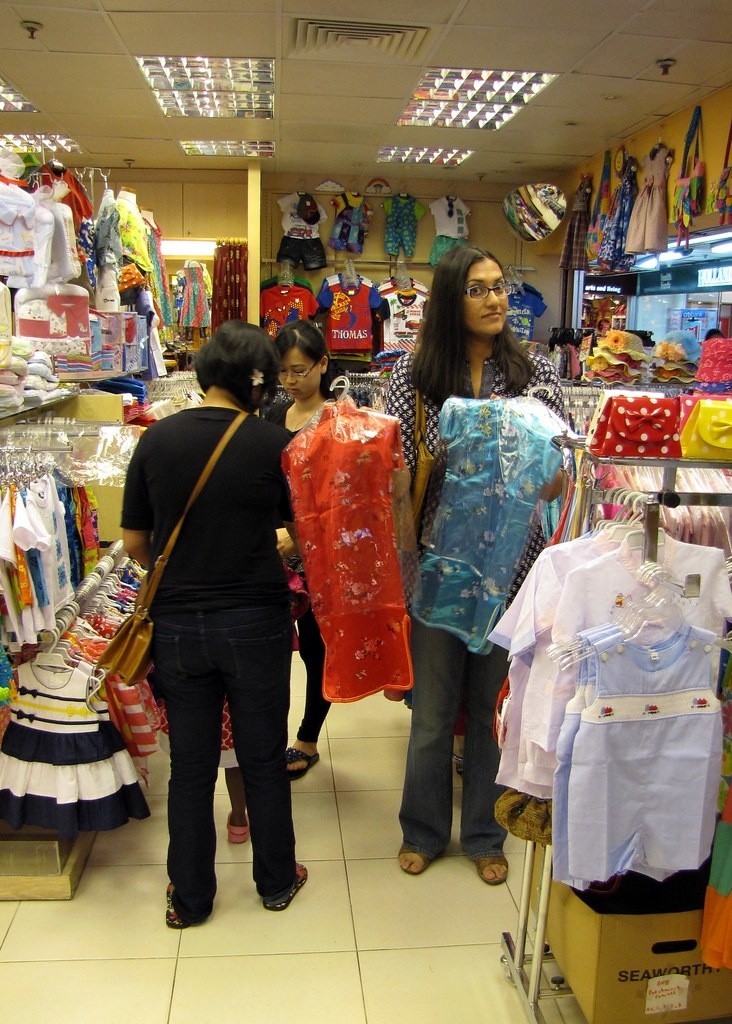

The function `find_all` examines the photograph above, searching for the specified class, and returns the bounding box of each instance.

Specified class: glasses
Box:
[277,362,320,381]
[463,283,514,300]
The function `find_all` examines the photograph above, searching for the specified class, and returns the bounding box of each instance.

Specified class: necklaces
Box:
[292,398,324,431]
[142,217,162,236]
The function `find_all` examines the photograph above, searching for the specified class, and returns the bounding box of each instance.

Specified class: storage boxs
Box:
[528,842,732,1024]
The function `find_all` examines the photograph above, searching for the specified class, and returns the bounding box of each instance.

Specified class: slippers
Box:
[264,862,308,912]
[286,747,320,782]
[474,855,510,886]
[163,882,192,930]
[226,809,249,843]
[397,844,446,875]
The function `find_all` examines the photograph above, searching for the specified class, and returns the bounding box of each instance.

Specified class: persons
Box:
[154,691,250,845]
[116,186,213,342]
[261,321,414,780]
[380,244,571,885]
[120,320,314,927]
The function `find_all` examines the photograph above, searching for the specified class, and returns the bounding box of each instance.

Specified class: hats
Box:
[582,328,732,393]
[296,195,321,225]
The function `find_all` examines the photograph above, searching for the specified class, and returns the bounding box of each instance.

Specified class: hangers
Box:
[330,177,373,213]
[0,142,202,706]
[276,259,525,400]
[525,327,732,671]
[575,173,591,197]
[380,180,414,207]
[641,125,672,164]
[428,183,471,216]
[616,149,638,177]
[277,176,307,204]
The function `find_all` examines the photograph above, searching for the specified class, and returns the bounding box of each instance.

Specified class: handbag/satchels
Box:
[705,120,731,225]
[494,789,553,847]
[678,400,731,465]
[677,395,731,440]
[387,349,433,552]
[118,264,145,293]
[95,556,171,683]
[582,395,667,453]
[668,104,703,251]
[588,395,683,459]
